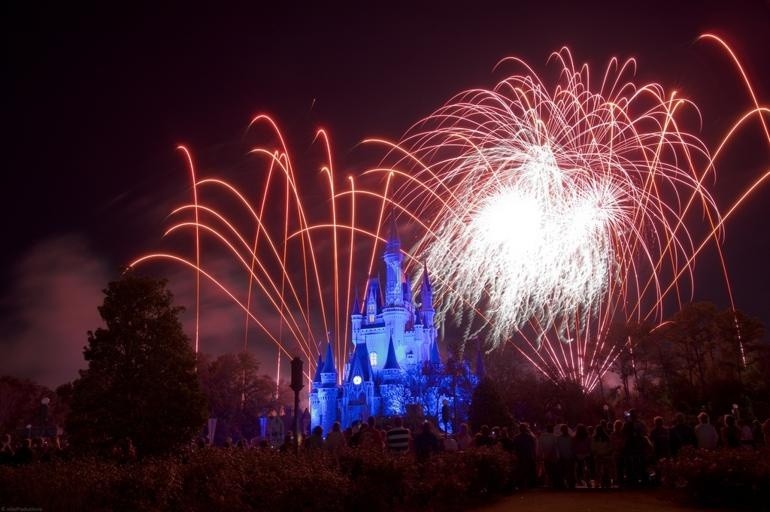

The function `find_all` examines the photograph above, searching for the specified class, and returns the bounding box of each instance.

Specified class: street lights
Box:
[287,355,307,449]
[24,396,50,439]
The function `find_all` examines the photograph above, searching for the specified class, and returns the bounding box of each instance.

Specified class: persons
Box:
[1,400,770,494]
